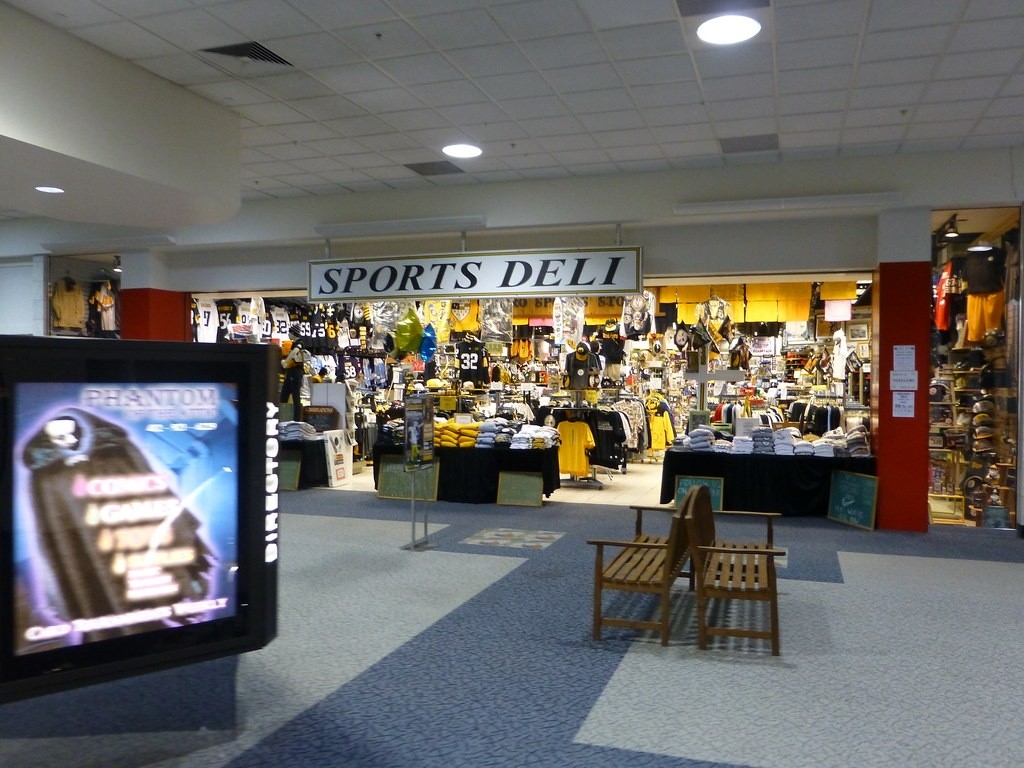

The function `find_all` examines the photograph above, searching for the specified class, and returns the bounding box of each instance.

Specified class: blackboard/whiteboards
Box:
[304,412,337,430]
[378,455,439,500]
[274,458,300,490]
[497,472,542,507]
[675,475,723,511]
[827,470,877,530]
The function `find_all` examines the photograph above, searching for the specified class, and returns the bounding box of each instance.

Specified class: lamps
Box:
[944,218,960,238]
[111,256,122,272]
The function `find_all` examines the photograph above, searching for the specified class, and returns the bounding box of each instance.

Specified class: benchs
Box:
[685,485,786,656]
[587,485,699,646]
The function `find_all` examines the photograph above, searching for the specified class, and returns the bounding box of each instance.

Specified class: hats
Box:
[973,402,997,415]
[972,427,995,440]
[972,389,994,401]
[972,414,994,427]
[972,440,994,452]
[576,342,589,356]
[427,378,445,387]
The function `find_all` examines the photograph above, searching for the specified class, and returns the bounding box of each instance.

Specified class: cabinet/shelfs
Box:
[929,369,981,494]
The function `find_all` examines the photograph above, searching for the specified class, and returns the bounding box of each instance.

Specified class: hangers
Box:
[701,296,731,308]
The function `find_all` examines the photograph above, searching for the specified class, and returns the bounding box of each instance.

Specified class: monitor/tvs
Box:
[0,334,281,704]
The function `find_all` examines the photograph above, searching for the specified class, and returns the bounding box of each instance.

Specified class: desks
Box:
[660,448,876,518]
[372,440,561,503]
